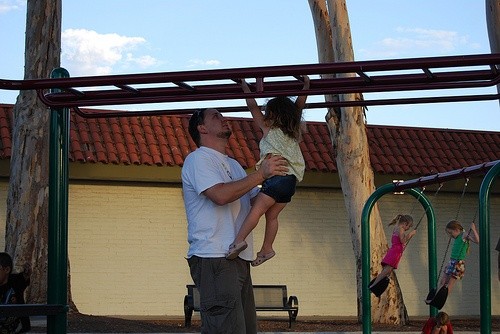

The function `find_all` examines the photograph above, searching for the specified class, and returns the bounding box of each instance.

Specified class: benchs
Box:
[184,285,299,329]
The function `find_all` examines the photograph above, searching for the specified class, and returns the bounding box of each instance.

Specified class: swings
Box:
[367,184,443,297]
[426,177,492,308]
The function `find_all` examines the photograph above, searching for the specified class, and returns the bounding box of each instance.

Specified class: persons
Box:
[423,221,479,303]
[369,214,417,288]
[181,108,289,334]
[225,74,309,266]
[421,312,453,334]
[0,252,30,334]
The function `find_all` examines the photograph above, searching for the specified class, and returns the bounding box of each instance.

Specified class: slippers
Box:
[225,241,248,259]
[251,251,275,266]
